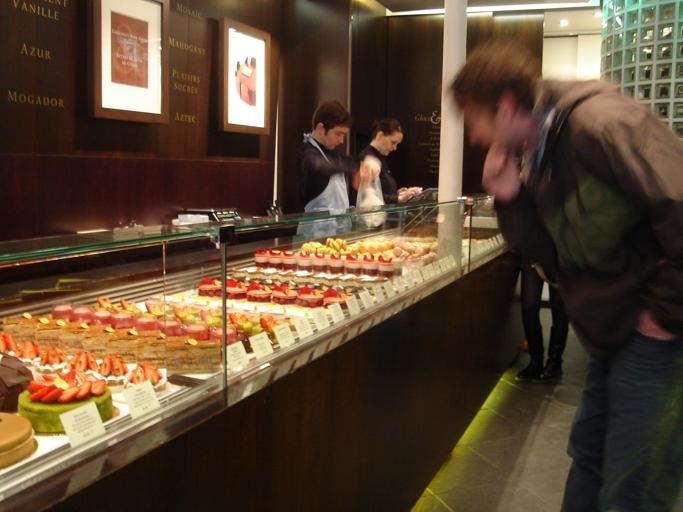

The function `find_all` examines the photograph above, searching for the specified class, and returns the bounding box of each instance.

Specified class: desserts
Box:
[0,237,438,469]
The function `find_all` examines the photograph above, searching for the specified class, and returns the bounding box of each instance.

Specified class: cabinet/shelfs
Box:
[1,188,531,511]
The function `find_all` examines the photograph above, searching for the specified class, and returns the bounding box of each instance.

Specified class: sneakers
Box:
[514,355,544,383]
[532,358,563,381]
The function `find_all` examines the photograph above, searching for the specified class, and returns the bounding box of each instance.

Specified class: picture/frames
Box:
[216,16,272,136]
[86,1,171,125]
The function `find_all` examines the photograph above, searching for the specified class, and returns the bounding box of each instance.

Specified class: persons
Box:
[293,97,382,241]
[511,262,568,384]
[446,36,683,512]
[357,113,422,205]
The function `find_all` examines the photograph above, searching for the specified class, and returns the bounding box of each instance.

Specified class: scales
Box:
[171,207,241,228]
